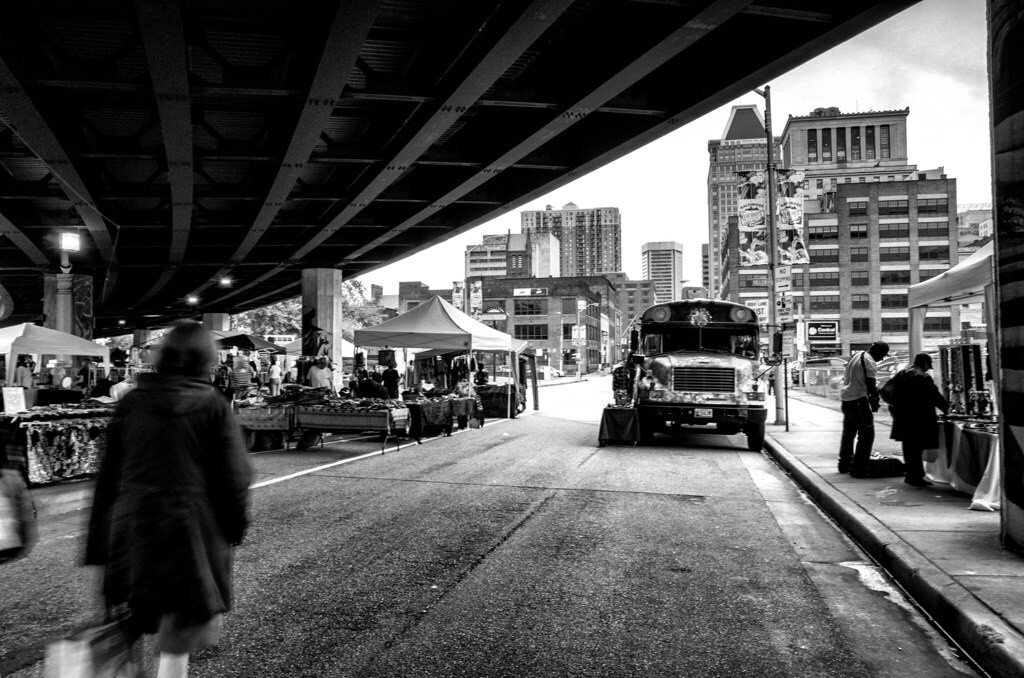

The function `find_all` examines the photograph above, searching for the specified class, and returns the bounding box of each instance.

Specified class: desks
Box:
[233,398,476,455]
[0,407,115,490]
[924,422,1000,501]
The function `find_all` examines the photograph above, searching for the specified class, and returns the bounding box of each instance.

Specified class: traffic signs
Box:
[772,265,792,294]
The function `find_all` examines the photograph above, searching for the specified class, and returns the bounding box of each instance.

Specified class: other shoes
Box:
[904,475,933,486]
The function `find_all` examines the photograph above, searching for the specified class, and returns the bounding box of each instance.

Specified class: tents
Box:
[907,239,1000,511]
[0,322,111,387]
[278,337,368,368]
[352,294,538,432]
[214,334,287,355]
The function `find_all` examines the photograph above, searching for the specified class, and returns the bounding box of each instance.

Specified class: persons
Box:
[381,359,402,399]
[219,353,234,386]
[229,355,256,389]
[16,355,36,374]
[768,372,776,395]
[474,363,488,385]
[281,358,291,383]
[268,359,283,396]
[358,366,368,381]
[404,360,414,387]
[838,340,890,474]
[371,365,384,386]
[48,320,256,678]
[306,357,333,390]
[889,353,950,486]
[72,358,91,394]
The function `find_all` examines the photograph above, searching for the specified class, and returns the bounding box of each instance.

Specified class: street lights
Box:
[555,311,563,372]
[577,302,600,378]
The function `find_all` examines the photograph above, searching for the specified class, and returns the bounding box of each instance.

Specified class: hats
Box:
[874,340,891,358]
[916,353,935,369]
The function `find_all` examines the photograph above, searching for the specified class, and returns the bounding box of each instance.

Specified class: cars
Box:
[792,350,941,398]
[482,365,566,378]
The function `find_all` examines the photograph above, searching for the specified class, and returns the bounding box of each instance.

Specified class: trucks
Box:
[630,299,784,453]
